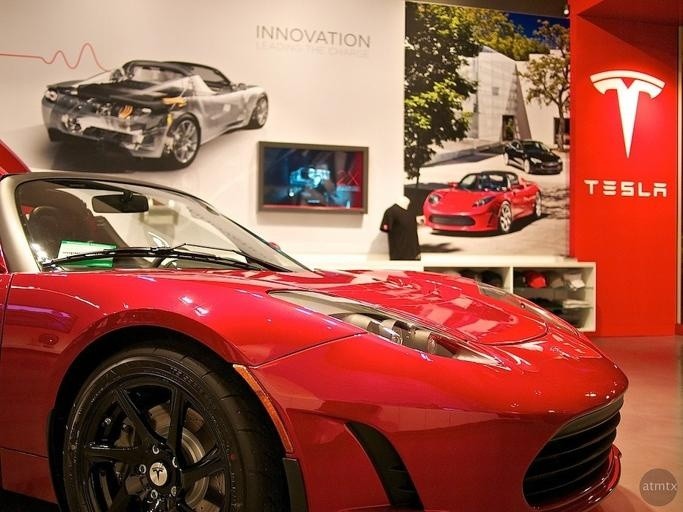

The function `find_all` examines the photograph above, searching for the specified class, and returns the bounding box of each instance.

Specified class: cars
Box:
[501,138,565,177]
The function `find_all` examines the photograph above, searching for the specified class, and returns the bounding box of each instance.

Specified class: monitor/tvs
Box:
[256,141,369,215]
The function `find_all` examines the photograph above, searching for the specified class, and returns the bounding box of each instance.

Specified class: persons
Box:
[380,196,421,260]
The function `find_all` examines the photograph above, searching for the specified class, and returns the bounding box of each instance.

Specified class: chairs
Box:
[27,206,141,270]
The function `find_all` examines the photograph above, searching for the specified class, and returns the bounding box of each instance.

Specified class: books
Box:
[562,299,591,309]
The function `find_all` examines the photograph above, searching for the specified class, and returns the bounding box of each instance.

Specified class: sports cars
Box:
[419,167,546,237]
[41,58,270,172]
[1,140,629,512]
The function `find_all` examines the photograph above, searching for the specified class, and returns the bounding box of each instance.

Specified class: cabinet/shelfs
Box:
[424,264,597,333]
